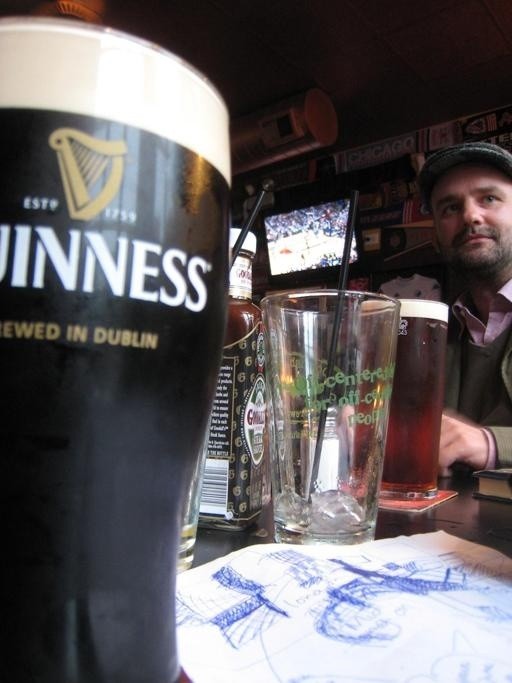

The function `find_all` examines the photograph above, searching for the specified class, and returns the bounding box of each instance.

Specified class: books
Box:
[469,466,511,502]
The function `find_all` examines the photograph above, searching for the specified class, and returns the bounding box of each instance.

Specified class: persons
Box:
[266,199,357,274]
[418,140,512,480]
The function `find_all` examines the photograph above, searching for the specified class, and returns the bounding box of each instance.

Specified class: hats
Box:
[413,142,512,209]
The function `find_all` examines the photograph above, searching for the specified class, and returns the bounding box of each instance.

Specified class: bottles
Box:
[195,227,271,532]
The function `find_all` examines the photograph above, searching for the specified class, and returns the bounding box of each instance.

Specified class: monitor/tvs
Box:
[260,191,362,284]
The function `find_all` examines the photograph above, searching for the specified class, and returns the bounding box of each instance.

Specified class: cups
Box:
[350,299,449,501]
[0,16,232,682]
[261,288,402,545]
